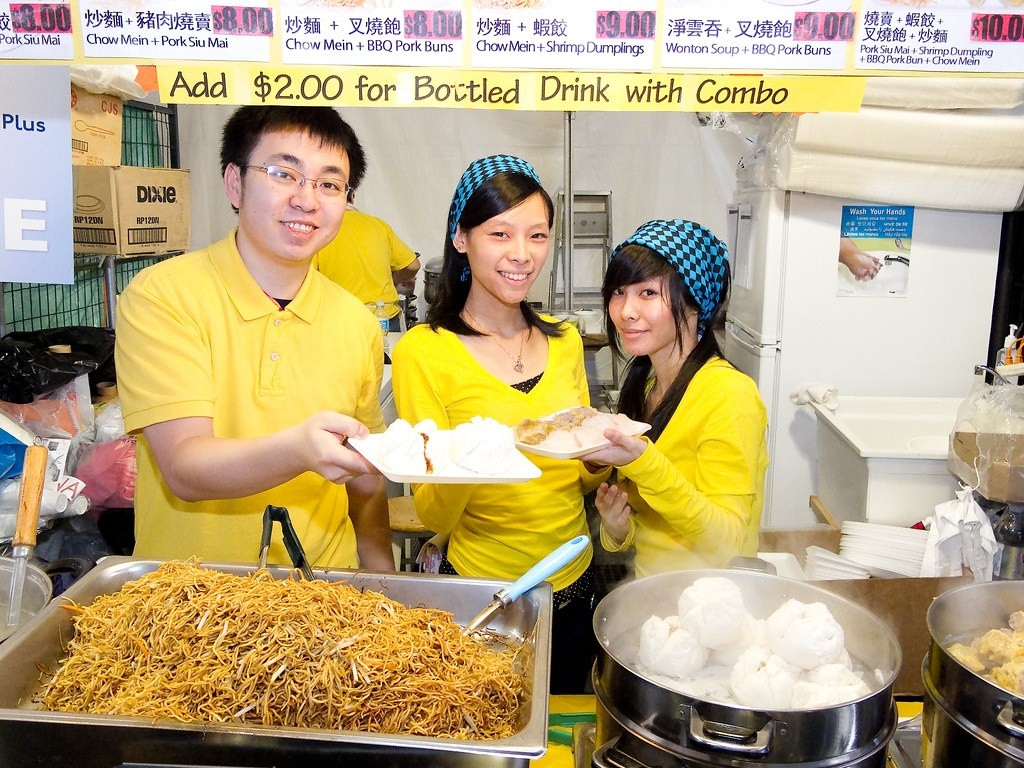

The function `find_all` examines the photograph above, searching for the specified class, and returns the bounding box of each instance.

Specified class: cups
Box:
[0,481,87,537]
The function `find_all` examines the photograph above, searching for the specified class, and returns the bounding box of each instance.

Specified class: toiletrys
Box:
[992,323,1019,387]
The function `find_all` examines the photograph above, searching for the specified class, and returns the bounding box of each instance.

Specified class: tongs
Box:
[547,712,597,746]
[892,712,921,741]
[254,503,316,581]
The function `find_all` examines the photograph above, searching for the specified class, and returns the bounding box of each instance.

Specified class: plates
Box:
[756,520,930,579]
[347,433,542,484]
[515,407,651,459]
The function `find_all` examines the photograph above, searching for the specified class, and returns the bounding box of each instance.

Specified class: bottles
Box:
[990,503,1024,581]
[373,302,390,354]
[49,345,91,427]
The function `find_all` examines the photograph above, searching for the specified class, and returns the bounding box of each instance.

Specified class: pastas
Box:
[20,554,542,740]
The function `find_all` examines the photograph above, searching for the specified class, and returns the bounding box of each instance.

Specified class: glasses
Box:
[243,164,354,205]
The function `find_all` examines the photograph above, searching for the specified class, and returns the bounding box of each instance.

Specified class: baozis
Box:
[628,576,874,709]
[374,415,515,477]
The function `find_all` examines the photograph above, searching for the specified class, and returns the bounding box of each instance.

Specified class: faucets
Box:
[974,364,1013,385]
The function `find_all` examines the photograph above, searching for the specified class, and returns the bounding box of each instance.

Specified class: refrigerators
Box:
[725,189,1004,527]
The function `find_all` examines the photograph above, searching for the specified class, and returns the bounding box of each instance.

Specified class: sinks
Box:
[807,395,968,532]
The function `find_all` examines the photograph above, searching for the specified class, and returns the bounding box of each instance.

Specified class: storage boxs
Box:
[0,552,565,768]
[948,431,1024,503]
[70,82,124,167]
[814,395,960,528]
[71,164,192,259]
[759,527,980,694]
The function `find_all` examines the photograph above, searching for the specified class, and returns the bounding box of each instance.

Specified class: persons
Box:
[113,106,396,574]
[594,218,767,580]
[306,201,421,333]
[390,152,613,696]
[894,238,910,251]
[838,228,881,281]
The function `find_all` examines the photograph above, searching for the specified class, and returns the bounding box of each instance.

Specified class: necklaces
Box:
[464,305,525,374]
[648,382,673,415]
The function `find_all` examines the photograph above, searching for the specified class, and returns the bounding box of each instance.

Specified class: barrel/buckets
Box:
[555,314,578,328]
[575,309,604,333]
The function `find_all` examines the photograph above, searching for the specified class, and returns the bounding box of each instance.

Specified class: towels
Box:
[788,381,841,412]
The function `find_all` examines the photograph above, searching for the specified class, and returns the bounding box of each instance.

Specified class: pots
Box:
[422,256,444,304]
[0,557,97,642]
[920,580,1024,768]
[592,555,901,768]
